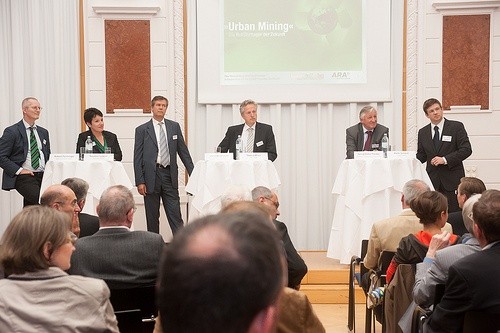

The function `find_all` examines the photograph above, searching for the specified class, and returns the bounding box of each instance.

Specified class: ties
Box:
[29,127,41,169]
[248,128,254,153]
[364,131,373,151]
[158,122,170,167]
[433,126,439,152]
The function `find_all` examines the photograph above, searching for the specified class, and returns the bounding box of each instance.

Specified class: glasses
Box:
[455,190,458,194]
[265,197,279,209]
[468,213,473,221]
[126,207,137,215]
[25,106,42,110]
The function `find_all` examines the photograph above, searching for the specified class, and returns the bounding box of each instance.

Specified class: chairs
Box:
[348,240,446,333]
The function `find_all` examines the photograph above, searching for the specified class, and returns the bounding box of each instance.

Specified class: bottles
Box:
[85,136,93,154]
[235,135,242,160]
[381,133,389,158]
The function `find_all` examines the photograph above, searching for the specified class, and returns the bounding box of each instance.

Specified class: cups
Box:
[80,147,87,161]
[104,146,111,154]
[215,147,221,153]
[372,144,380,151]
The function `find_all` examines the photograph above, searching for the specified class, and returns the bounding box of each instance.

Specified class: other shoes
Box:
[367,287,384,309]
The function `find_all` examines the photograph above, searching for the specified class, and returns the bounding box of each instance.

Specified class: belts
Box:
[156,163,171,168]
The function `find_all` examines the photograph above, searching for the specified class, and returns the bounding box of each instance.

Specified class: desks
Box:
[38,160,133,216]
[326,157,435,265]
[185,159,282,224]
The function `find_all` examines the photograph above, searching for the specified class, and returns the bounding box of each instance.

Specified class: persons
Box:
[0,178,325,333]
[133,96,195,237]
[0,97,51,209]
[345,104,389,160]
[76,108,123,161]
[417,99,472,194]
[364,177,500,333]
[216,98,277,162]
